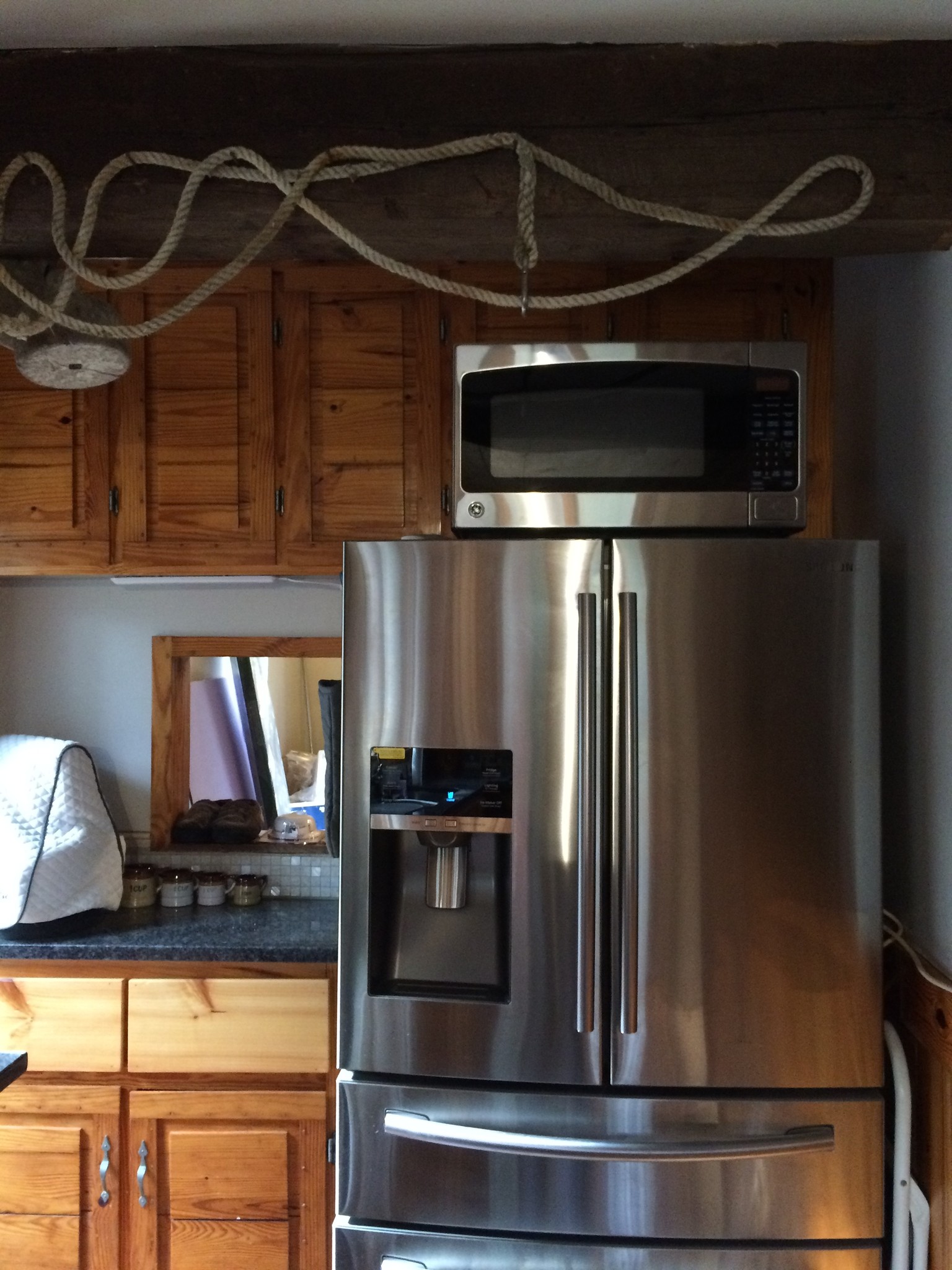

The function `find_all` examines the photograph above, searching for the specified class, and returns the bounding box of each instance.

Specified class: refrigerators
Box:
[330,534,884,1270]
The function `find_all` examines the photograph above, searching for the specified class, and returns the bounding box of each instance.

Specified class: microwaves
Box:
[453,339,809,531]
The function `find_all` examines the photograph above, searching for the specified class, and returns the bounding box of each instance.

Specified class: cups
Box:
[233,874,268,905]
[158,867,197,907]
[197,872,237,906]
[120,863,163,908]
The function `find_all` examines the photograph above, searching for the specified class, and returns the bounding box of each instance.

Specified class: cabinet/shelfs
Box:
[0,959,336,1270]
[0,265,271,577]
[282,265,610,575]
[633,258,835,540]
